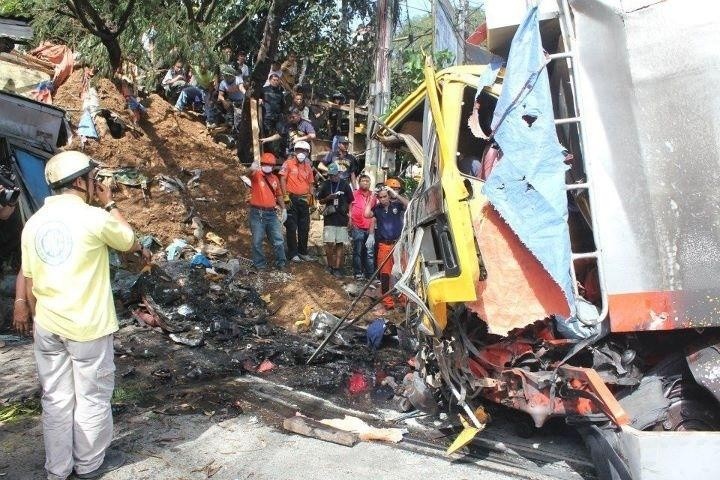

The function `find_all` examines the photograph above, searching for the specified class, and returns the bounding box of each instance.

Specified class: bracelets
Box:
[104,199,118,213]
[14,298,27,306]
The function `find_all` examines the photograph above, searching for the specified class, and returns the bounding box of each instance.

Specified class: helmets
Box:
[384,178,402,188]
[294,140,311,154]
[43,150,100,190]
[260,152,276,165]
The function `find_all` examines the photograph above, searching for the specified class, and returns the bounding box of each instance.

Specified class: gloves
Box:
[249,160,259,172]
[279,208,288,224]
[364,233,376,248]
[283,193,292,206]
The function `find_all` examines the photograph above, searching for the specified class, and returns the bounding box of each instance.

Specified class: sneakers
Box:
[382,295,396,311]
[291,255,301,263]
[325,264,333,274]
[355,272,365,281]
[297,252,317,262]
[332,267,343,278]
[76,451,126,479]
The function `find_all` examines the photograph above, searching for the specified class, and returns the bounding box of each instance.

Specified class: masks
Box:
[296,153,307,164]
[261,165,273,174]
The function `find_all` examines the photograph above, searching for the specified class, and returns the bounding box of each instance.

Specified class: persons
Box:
[1,164,21,228]
[12,267,31,339]
[23,150,143,480]
[158,45,411,313]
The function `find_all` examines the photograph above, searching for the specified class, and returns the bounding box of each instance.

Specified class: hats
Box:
[338,135,351,143]
[327,162,339,175]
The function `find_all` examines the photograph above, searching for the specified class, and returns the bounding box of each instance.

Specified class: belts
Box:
[251,205,277,211]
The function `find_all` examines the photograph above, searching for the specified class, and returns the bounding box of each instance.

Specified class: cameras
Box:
[0,165,20,207]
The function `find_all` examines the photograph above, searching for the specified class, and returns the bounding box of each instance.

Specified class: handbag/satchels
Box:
[306,192,314,207]
